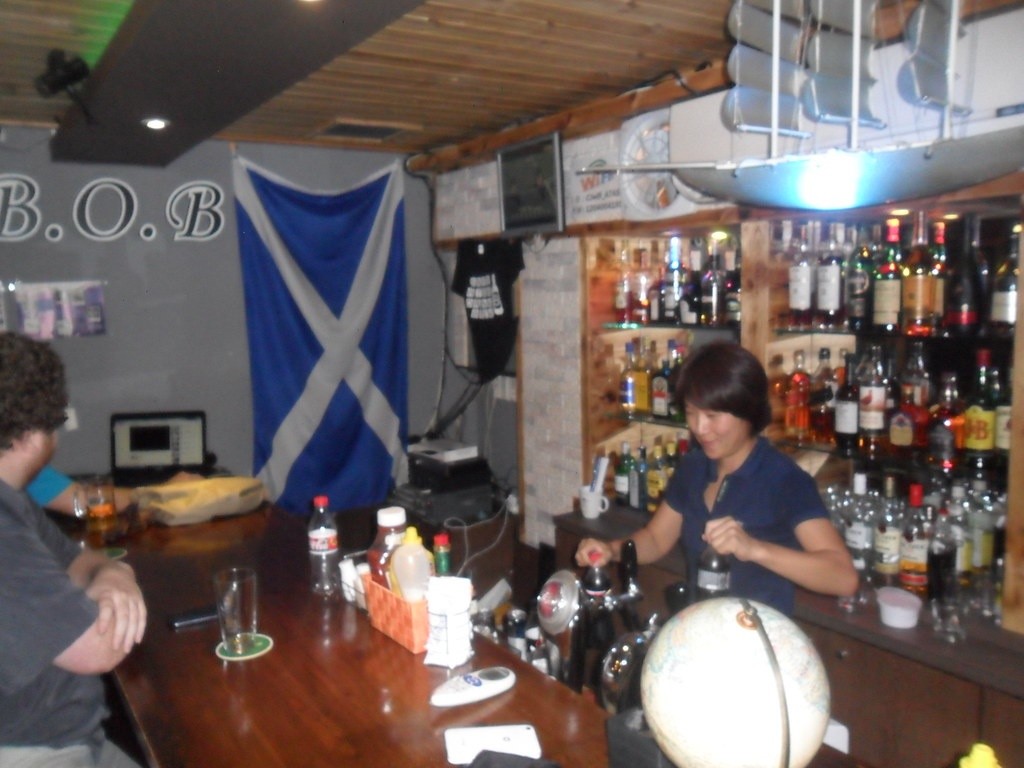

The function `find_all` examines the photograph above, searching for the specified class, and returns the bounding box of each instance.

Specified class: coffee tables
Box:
[397,508,518,599]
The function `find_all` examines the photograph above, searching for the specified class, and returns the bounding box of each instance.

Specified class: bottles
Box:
[307,496,342,601]
[389,526,437,602]
[368,506,406,591]
[432,534,454,578]
[580,552,614,649]
[601,211,1022,620]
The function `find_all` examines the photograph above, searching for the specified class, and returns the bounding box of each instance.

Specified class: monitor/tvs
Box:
[497,130,565,239]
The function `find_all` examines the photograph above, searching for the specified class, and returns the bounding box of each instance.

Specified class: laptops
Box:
[104,410,210,489]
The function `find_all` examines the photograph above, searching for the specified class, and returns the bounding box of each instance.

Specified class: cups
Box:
[875,585,924,629]
[74,474,120,544]
[213,567,259,657]
[930,593,965,644]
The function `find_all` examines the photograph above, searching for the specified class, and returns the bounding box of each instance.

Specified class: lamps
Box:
[36,50,90,121]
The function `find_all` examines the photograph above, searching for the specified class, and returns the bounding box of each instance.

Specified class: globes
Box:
[640,595,832,768]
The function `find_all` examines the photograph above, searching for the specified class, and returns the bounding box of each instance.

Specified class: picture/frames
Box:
[497,132,564,236]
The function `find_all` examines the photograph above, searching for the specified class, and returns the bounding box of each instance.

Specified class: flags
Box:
[726,44,800,97]
[800,76,888,130]
[902,1,969,67]
[720,85,807,139]
[727,0,803,63]
[896,53,973,119]
[747,0,804,22]
[810,0,878,38]
[802,30,876,84]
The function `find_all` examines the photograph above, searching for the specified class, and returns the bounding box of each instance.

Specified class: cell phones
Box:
[444,724,542,766]
[167,607,219,629]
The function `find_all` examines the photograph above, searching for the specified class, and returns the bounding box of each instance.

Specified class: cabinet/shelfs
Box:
[581,172,1021,641]
[551,496,1023,768]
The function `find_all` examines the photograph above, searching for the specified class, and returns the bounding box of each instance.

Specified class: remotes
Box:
[432,666,517,709]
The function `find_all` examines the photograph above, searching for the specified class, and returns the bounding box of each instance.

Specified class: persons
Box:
[0,331,146,768]
[575,339,860,622]
[24,464,208,518]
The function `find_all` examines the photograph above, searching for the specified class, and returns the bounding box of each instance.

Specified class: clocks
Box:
[620,107,696,219]
